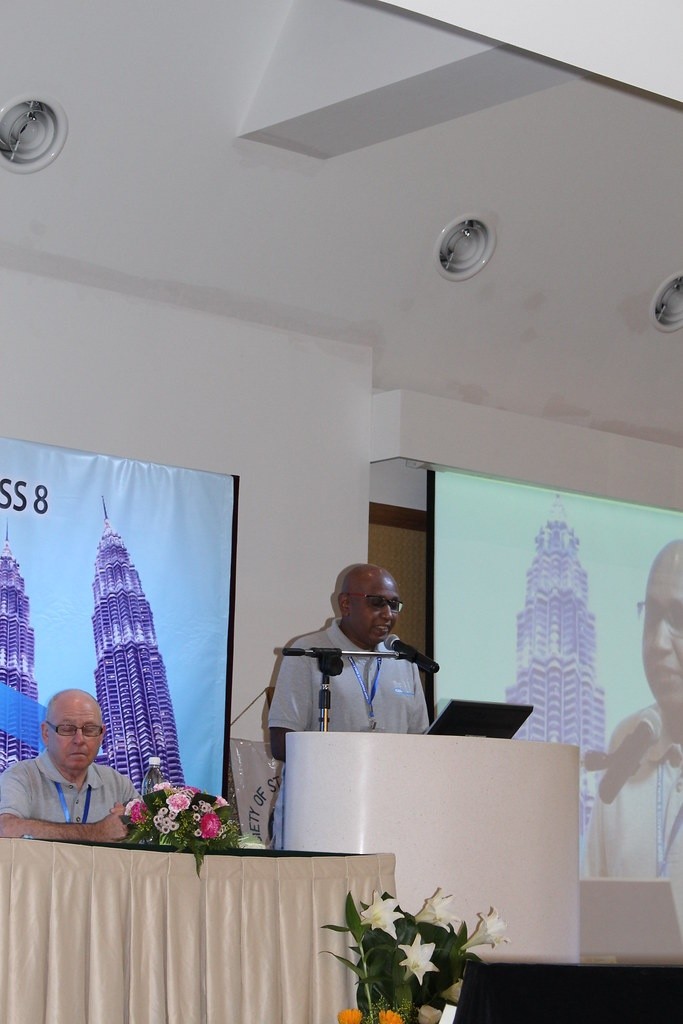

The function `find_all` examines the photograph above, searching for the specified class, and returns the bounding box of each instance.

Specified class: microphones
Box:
[600,708,661,804]
[385,635,440,676]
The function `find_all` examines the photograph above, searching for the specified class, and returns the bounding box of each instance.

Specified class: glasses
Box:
[350,592,404,613]
[637,602,683,638]
[46,720,103,737]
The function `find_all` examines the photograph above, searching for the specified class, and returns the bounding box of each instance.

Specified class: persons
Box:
[0,689,142,842]
[585,539,683,882]
[268,564,429,761]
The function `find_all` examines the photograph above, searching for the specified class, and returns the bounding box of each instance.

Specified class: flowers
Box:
[320,885,510,1024]
[115,781,243,880]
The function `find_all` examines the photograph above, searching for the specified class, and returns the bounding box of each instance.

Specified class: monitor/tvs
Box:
[421,699,535,740]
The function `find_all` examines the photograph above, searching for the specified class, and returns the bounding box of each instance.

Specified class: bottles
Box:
[142,757,166,796]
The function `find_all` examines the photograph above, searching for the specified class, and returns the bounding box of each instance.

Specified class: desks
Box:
[0,838,395,1024]
